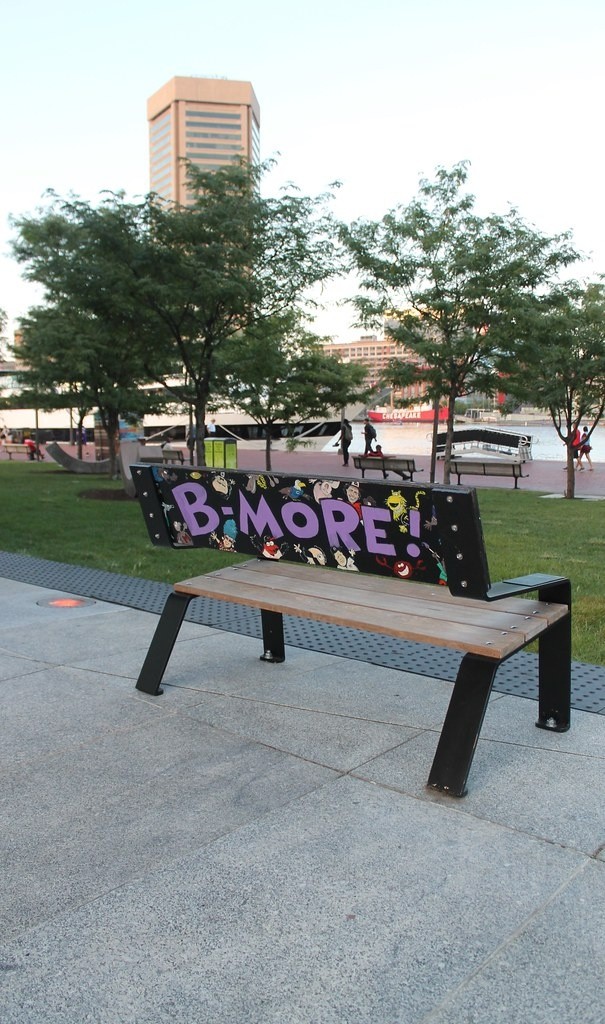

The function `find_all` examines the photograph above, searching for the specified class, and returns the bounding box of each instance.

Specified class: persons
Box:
[562,422,594,471]
[207,418,216,436]
[160,436,174,463]
[186,424,196,450]
[361,419,377,454]
[25,435,45,459]
[1,425,13,444]
[359,445,411,480]
[336,419,351,466]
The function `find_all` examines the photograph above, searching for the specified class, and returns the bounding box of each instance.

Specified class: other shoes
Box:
[562,467,567,470]
[342,463,348,467]
[578,468,585,472]
[587,469,593,471]
[573,468,577,471]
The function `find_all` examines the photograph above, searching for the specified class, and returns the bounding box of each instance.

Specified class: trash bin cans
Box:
[10,429,53,443]
[204,437,238,469]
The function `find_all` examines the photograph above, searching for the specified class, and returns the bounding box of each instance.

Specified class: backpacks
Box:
[366,425,376,438]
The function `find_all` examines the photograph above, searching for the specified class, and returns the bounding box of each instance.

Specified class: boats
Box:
[366,385,450,423]
[0,361,395,453]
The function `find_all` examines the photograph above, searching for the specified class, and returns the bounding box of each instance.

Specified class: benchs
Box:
[3,444,34,460]
[162,450,190,465]
[448,461,530,490]
[351,456,425,482]
[130,463,575,799]
